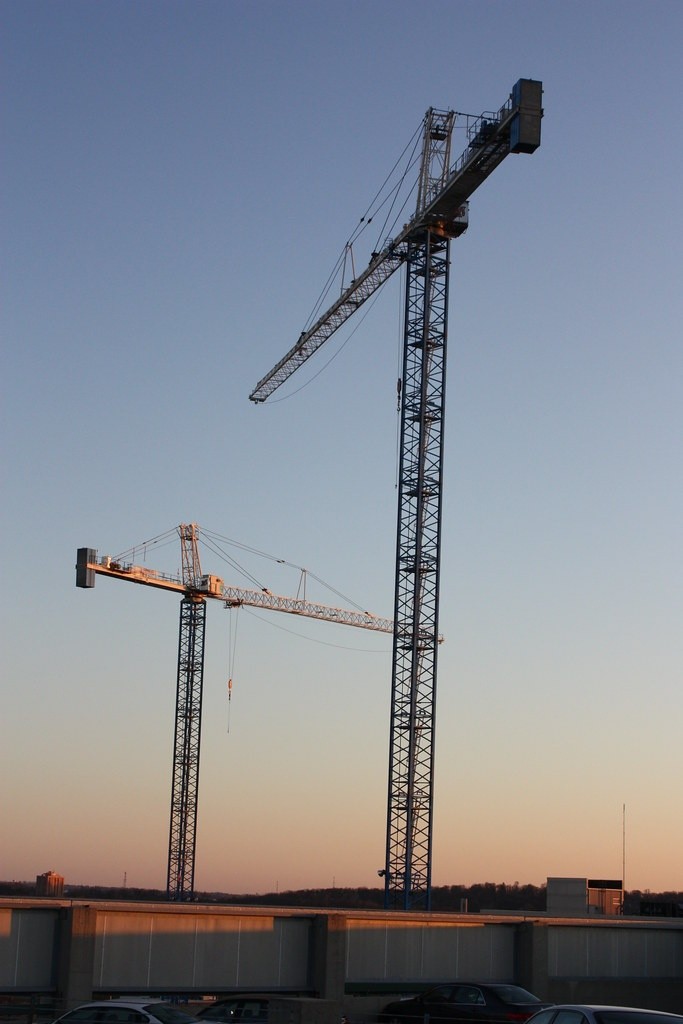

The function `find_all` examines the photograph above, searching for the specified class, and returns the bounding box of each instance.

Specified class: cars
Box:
[377,980,555,1024]
[193,994,290,1024]
[49,996,229,1024]
[523,1004,683,1024]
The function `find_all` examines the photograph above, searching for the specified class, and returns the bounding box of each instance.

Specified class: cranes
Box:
[247,79,545,912]
[76,521,446,900]
